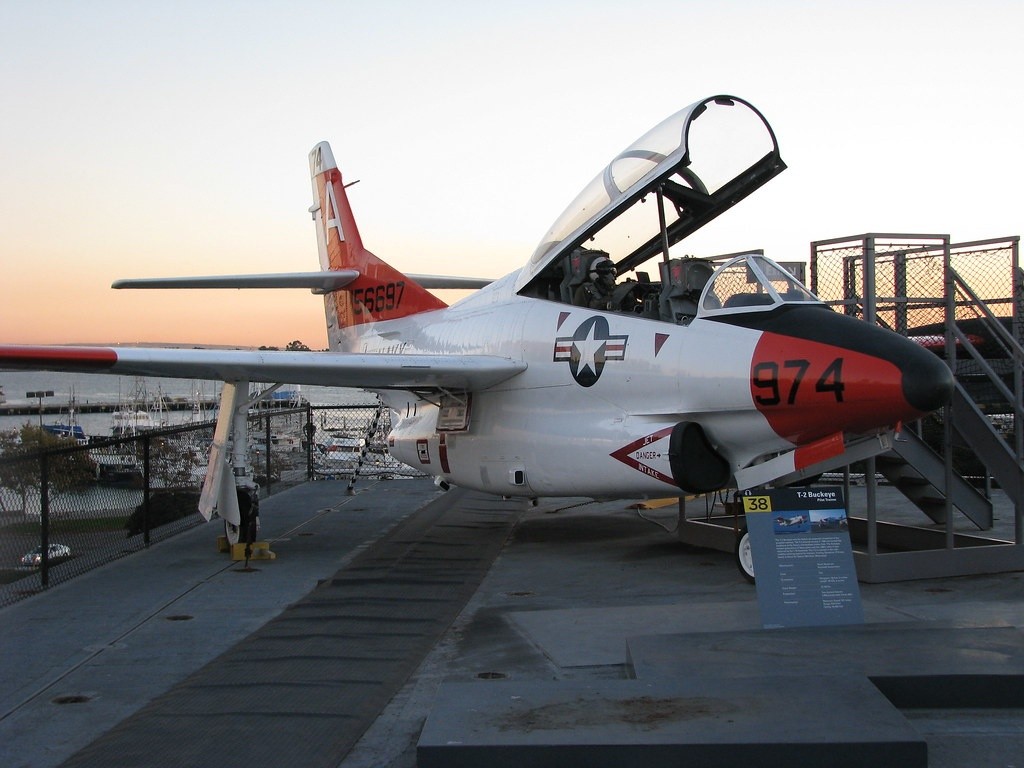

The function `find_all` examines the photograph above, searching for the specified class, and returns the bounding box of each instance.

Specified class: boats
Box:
[63,452,142,483]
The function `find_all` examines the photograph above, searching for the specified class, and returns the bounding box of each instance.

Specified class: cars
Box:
[22,543,71,568]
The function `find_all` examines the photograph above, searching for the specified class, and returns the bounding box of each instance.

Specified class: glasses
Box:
[596,266,617,276]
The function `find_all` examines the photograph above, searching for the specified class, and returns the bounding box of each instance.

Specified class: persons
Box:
[574,257,617,312]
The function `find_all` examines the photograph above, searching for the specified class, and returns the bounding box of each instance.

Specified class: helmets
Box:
[588,257,617,284]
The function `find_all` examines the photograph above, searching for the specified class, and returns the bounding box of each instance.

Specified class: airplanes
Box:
[0,94,988,589]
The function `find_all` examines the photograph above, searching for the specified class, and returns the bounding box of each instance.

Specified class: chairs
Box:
[659,258,721,325]
[560,250,610,304]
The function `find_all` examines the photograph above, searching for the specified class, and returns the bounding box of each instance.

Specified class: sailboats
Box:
[42,384,86,440]
[109,377,431,495]
